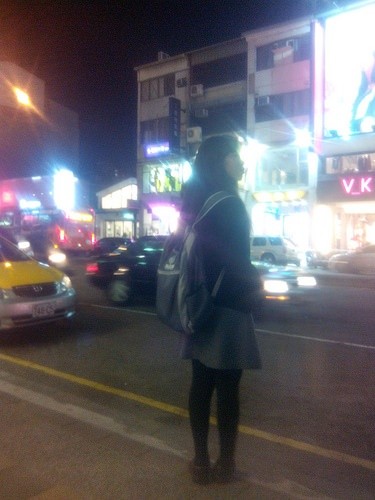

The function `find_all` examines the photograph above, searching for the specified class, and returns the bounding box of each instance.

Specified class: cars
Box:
[0,222,70,274]
[85,236,128,264]
[84,234,322,313]
[0,233,78,339]
[248,235,303,268]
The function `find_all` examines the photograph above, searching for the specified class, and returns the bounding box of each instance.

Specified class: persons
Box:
[350,48,375,135]
[176,133,266,485]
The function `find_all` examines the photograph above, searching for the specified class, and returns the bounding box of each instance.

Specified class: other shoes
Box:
[212,459,236,485]
[188,456,210,485]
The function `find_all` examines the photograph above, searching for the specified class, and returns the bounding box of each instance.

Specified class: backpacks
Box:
[155,191,238,337]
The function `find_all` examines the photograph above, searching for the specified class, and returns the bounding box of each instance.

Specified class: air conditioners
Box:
[189,84,204,98]
[193,108,209,119]
[258,97,269,107]
[285,39,298,52]
[186,126,203,144]
